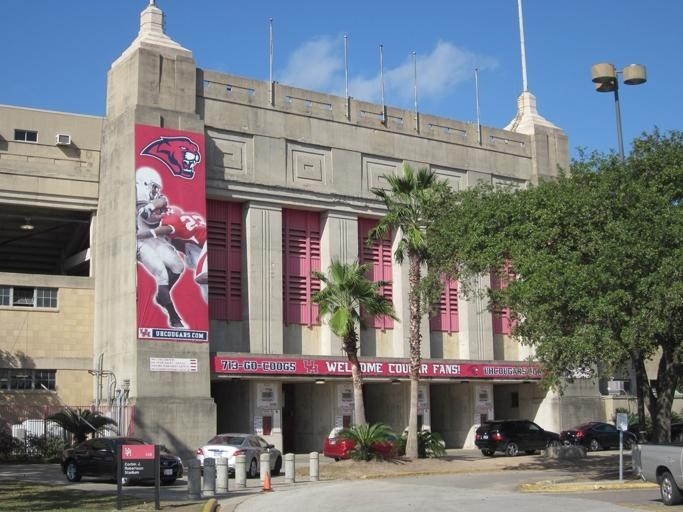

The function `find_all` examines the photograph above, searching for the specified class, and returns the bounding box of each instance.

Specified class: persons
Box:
[135,165,187,329]
[136,203,208,306]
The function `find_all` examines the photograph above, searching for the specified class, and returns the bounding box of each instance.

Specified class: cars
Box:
[196,432,282,478]
[61,437,184,485]
[561,422,638,451]
[323,426,394,462]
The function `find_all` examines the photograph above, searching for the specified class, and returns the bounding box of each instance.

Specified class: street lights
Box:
[590,63,648,444]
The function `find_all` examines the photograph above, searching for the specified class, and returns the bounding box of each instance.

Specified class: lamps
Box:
[20,217,34,231]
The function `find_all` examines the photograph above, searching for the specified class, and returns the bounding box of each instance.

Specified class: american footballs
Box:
[140,208,171,226]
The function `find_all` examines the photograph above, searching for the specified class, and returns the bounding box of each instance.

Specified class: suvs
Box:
[475,419,561,457]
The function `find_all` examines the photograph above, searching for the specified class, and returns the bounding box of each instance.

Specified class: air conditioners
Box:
[56,134,72,146]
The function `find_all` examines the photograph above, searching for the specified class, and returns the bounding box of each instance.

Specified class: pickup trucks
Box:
[631,442,683,505]
